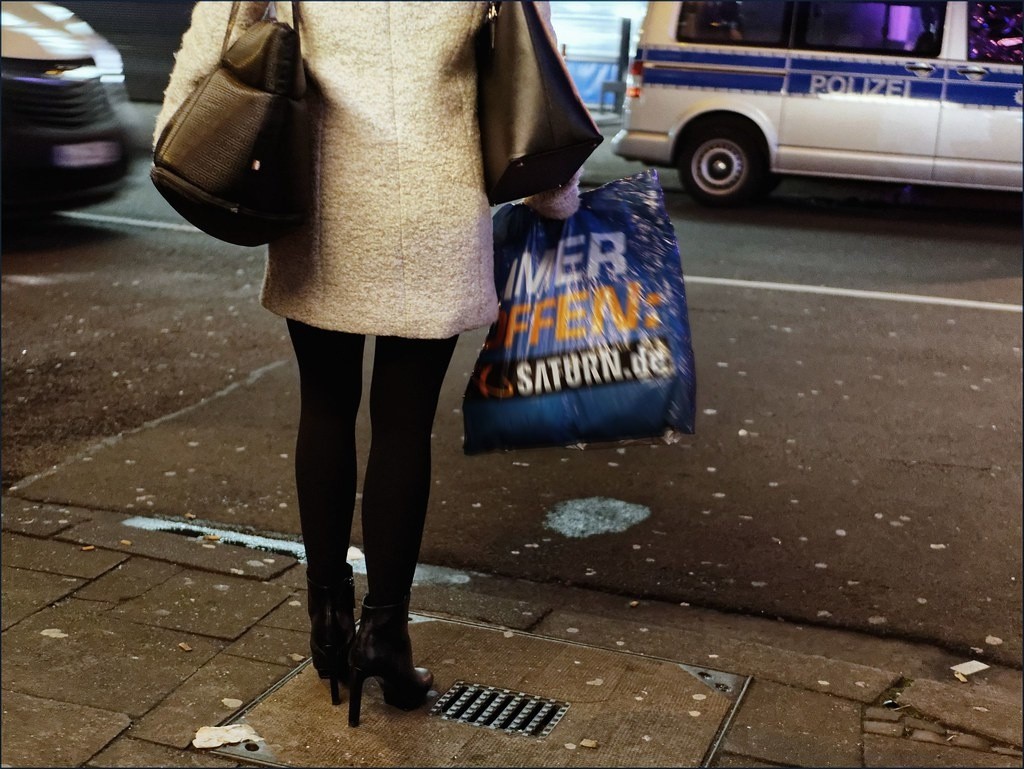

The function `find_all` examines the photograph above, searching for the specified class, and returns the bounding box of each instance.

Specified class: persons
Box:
[153,2,583,735]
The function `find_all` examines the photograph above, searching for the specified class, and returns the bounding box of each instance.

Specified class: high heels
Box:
[306,563,358,704]
[348,593,434,728]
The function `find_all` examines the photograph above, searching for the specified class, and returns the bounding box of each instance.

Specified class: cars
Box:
[0,2,143,217]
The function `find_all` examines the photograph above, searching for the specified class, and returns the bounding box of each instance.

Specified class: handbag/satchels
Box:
[150,2,308,247]
[476,1,603,206]
[460,168,697,457]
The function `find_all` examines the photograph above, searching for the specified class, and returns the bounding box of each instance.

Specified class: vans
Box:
[609,0,1023,208]
